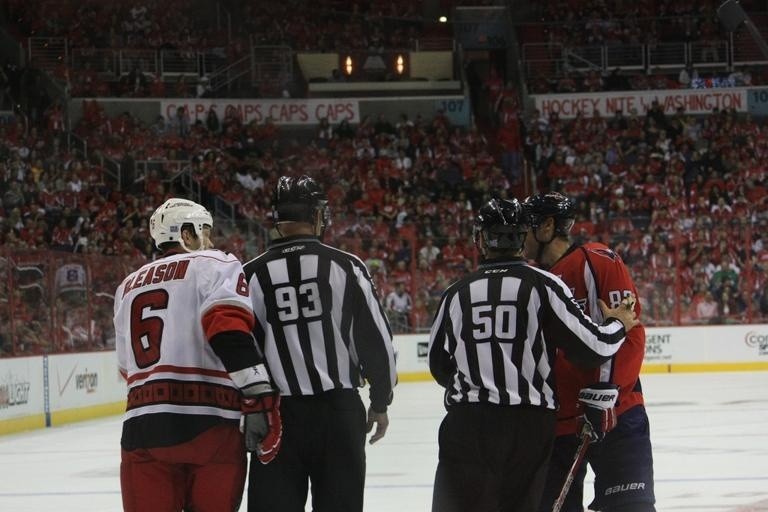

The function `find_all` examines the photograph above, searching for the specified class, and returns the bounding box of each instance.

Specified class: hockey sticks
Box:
[63,326,75,351]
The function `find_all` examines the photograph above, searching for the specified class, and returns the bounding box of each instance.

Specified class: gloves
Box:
[237,388,286,464]
[575,384,622,446]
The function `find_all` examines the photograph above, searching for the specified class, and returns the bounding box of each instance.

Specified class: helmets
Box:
[149,196,214,251]
[272,170,330,225]
[524,189,578,230]
[473,197,529,241]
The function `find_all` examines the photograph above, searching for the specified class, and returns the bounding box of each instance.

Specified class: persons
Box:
[111,198,284,512]
[244,172,400,512]
[425,198,643,512]
[524,190,657,512]
[0,0,766,359]
